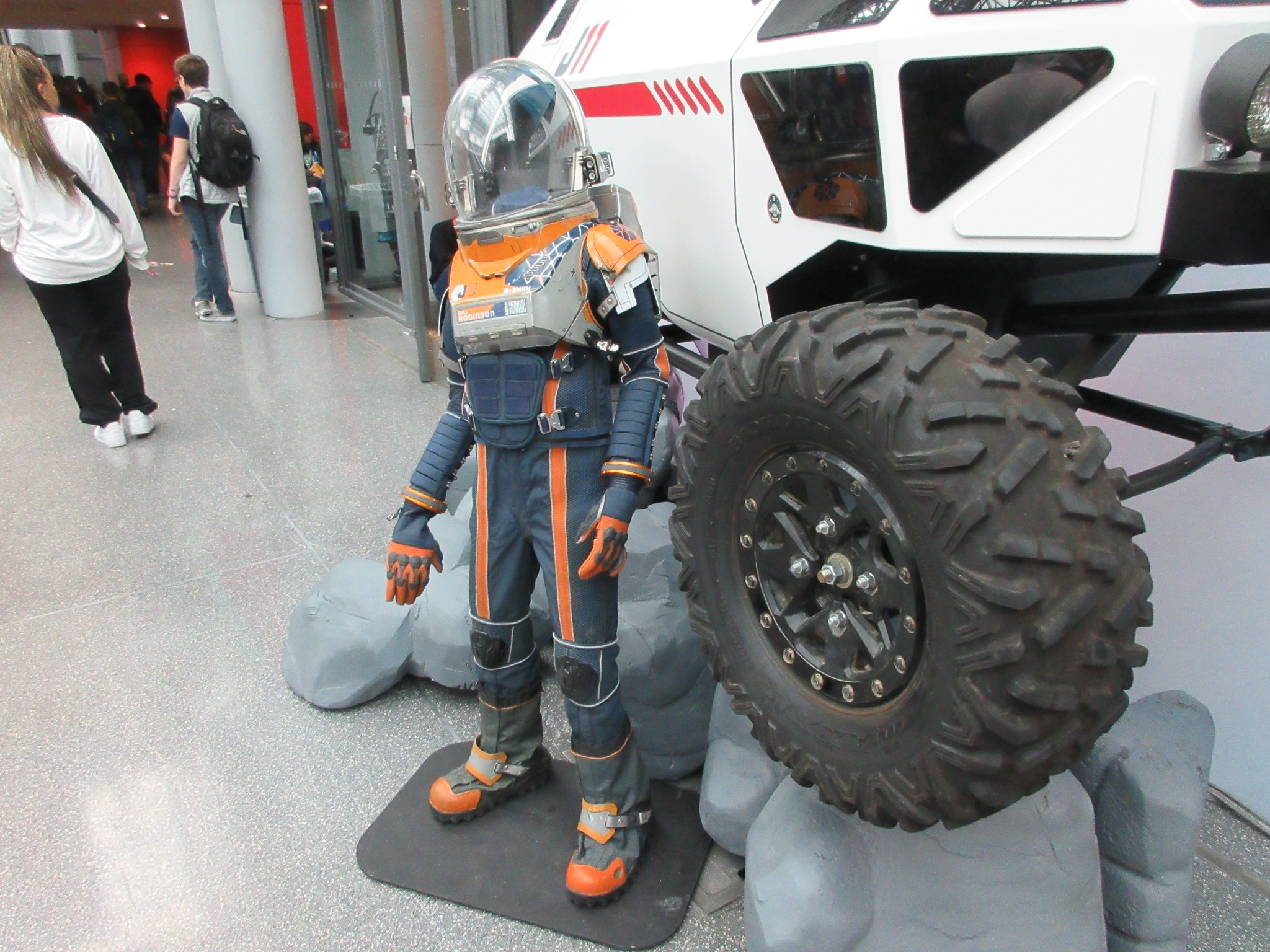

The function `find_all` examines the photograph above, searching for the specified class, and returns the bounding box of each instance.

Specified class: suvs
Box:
[517,0,1270,845]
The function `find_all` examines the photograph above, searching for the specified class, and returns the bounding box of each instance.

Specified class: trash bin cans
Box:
[348,182,399,279]
[219,190,259,293]
[307,186,327,298]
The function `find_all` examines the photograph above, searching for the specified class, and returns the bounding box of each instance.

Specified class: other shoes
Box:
[564,710,656,909]
[428,680,554,824]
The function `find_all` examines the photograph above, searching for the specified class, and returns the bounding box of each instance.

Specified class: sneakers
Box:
[94,418,127,448]
[138,204,150,217]
[199,305,237,322]
[195,300,211,317]
[127,409,155,435]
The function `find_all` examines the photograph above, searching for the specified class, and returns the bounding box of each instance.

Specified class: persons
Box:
[129,74,169,199]
[386,58,672,908]
[96,81,150,217]
[0,44,159,448]
[76,77,101,114]
[63,75,95,125]
[116,73,131,104]
[169,53,240,323]
[298,121,323,170]
[53,74,76,121]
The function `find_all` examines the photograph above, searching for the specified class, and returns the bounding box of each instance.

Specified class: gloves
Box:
[384,498,445,606]
[573,472,645,580]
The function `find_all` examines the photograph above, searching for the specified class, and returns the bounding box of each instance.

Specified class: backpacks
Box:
[182,96,261,246]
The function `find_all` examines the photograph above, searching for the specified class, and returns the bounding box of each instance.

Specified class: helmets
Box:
[441,56,616,224]
